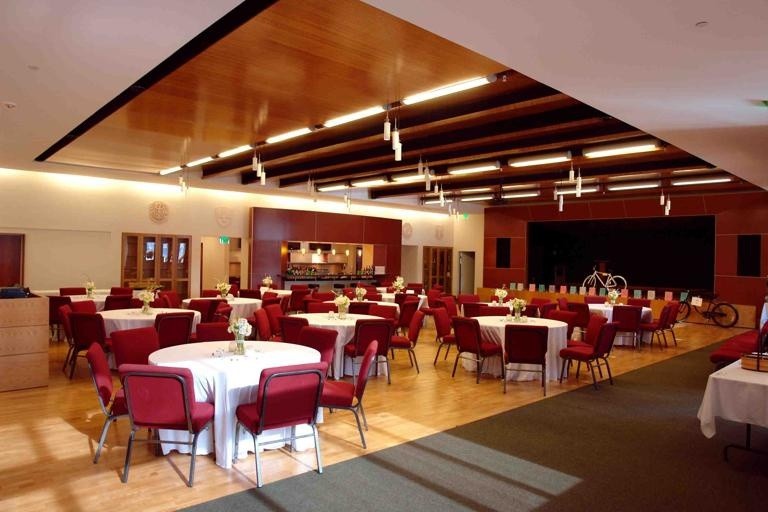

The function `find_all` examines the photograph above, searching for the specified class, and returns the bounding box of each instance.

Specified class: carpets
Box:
[176,330,768,512]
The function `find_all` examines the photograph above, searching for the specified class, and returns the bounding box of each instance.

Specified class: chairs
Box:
[86,342,129,463]
[708,295,768,363]
[255,282,423,388]
[47,282,261,379]
[319,340,379,450]
[115,364,216,488]
[420,285,681,397]
[236,364,328,491]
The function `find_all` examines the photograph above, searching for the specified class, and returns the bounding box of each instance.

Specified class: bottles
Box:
[363,266,373,275]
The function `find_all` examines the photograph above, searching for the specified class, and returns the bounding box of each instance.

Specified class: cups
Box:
[229,342,236,352]
[492,301,496,305]
[329,311,334,318]
[217,295,221,298]
[522,316,527,323]
[507,314,512,320]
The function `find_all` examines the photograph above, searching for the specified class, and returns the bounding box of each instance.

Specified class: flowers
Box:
[261,274,273,287]
[392,276,405,290]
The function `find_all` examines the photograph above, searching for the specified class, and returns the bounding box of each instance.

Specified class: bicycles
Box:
[579,265,627,297]
[674,289,740,329]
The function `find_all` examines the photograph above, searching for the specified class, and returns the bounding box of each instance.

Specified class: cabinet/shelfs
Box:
[422,245,453,297]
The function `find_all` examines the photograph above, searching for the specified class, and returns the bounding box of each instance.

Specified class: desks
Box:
[696,351,768,463]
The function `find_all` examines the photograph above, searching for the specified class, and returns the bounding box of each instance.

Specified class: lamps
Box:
[317,243,321,256]
[345,245,349,256]
[583,141,658,159]
[331,242,335,255]
[509,152,571,169]
[301,241,306,256]
[501,168,735,218]
[358,246,362,256]
